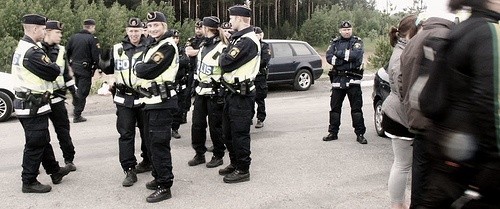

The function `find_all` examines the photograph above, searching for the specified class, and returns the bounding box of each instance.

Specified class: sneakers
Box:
[122,169,137,185]
[136,161,153,172]
[323,133,338,141]
[172,130,181,138]
[255,121,263,128]
[146,179,160,190]
[66,160,76,170]
[205,156,223,167]
[219,163,250,182]
[188,155,205,166]
[146,187,171,201]
[50,164,72,183]
[22,180,52,193]
[357,135,367,144]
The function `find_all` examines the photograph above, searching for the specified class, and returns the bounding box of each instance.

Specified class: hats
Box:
[171,29,180,37]
[147,12,167,22]
[126,17,142,27]
[228,5,251,17]
[46,21,64,30]
[254,27,262,33]
[339,22,352,28]
[83,19,96,25]
[23,14,47,25]
[194,17,231,29]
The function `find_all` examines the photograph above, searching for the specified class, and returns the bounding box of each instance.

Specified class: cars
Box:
[0,71,15,122]
[263,40,323,91]
[373,68,390,137]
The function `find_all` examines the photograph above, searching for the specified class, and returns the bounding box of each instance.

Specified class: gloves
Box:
[100,45,111,59]
[71,93,82,105]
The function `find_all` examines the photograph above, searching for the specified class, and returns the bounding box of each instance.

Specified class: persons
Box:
[11,14,72,193]
[99,17,152,186]
[67,19,100,122]
[170,29,190,139]
[398,0,500,209]
[219,7,261,183]
[132,11,179,203]
[35,21,77,171]
[185,20,204,72]
[251,27,271,128]
[381,15,415,209]
[188,16,226,167]
[322,21,366,144]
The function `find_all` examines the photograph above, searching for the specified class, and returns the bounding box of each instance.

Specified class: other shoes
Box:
[206,145,226,152]
[73,117,86,122]
